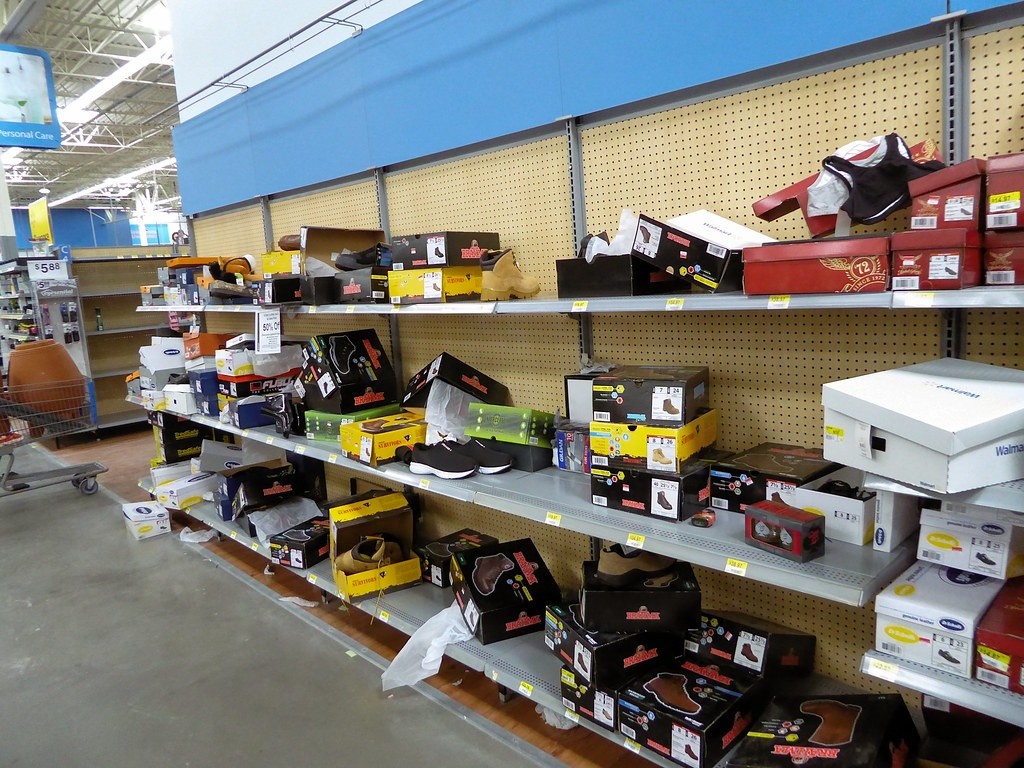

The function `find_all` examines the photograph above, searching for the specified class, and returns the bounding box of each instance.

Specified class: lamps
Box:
[38,184,51,194]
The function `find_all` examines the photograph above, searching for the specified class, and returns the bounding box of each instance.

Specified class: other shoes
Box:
[335,241,392,270]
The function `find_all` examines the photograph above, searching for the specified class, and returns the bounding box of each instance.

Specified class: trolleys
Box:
[0,373,109,503]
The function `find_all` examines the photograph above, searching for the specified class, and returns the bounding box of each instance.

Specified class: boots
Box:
[435,247,443,257]
[433,283,440,291]
[658,491,672,509]
[663,399,679,414]
[652,448,672,464]
[640,226,651,243]
[480,248,541,301]
[335,536,402,625]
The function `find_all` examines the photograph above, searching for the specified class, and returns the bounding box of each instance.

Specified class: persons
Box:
[0,340,24,444]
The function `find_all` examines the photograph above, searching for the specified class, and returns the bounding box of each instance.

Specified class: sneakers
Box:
[443,438,513,474]
[409,443,478,479]
[597,543,677,586]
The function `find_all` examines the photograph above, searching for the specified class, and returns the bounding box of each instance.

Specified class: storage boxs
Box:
[123,138,1024,768]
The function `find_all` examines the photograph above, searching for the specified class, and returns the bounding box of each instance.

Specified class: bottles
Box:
[95,307,103,331]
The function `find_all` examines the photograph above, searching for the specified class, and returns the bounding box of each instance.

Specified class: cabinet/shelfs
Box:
[0,256,102,453]
[124,0,1024,768]
[69,244,190,428]
